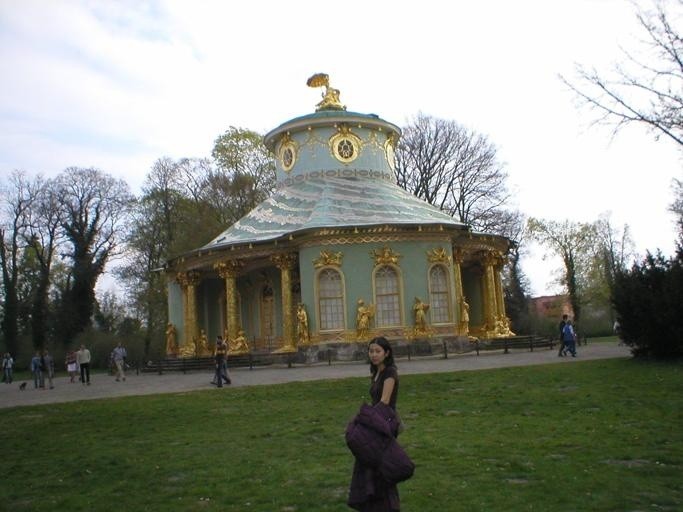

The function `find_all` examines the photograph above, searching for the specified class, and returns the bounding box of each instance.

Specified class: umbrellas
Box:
[306,72,329,95]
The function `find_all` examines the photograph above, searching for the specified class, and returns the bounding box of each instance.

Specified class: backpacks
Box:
[564,325,573,343]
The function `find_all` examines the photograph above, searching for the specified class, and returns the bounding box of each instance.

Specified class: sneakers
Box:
[210,379,233,388]
[1,378,14,384]
[558,350,577,358]
[35,377,126,390]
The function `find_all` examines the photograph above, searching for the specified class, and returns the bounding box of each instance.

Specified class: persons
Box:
[369,337,399,410]
[165,320,250,358]
[296,304,308,343]
[614,319,624,346]
[461,295,469,334]
[558,313,578,357]
[413,296,431,331]
[212,336,230,388]
[357,300,369,339]
[1,342,128,390]
[316,85,339,106]
